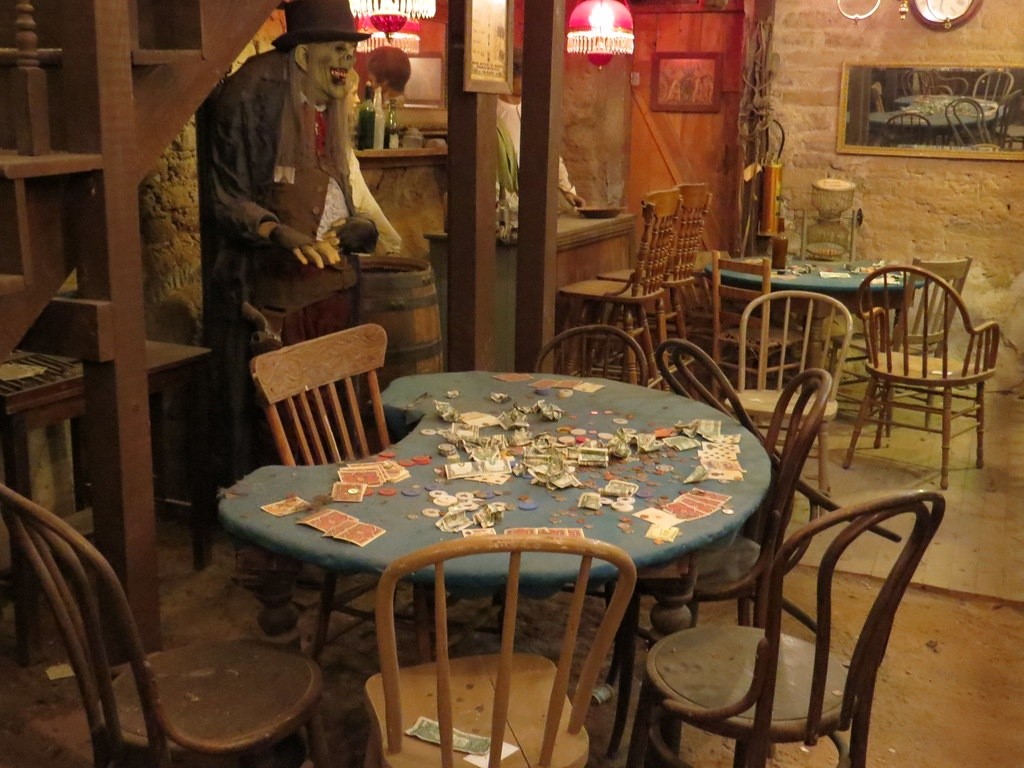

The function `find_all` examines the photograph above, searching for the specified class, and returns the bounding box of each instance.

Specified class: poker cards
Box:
[630,486,734,527]
[330,458,412,503]
[259,495,387,548]
[461,526,586,539]
[696,434,746,481]
[491,372,605,394]
[464,474,511,485]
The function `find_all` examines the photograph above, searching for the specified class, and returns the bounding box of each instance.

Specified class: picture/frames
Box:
[463,0,514,95]
[650,51,723,113]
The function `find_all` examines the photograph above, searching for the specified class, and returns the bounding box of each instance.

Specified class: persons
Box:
[494,47,585,211]
[367,47,411,108]
[196,1,401,489]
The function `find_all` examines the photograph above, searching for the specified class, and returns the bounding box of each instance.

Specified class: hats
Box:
[271,0,371,50]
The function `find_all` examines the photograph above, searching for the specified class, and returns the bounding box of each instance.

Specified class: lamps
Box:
[349,0,437,19]
[355,13,424,55]
[566,0,634,66]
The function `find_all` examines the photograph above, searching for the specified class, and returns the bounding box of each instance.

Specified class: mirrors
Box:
[836,59,1024,162]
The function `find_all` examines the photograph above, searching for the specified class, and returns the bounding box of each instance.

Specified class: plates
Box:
[578,207,624,218]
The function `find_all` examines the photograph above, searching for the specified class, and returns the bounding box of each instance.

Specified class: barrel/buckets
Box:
[279,253,446,462]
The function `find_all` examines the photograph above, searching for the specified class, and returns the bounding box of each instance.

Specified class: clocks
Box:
[907,0,982,31]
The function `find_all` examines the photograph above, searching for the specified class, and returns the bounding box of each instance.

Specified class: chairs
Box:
[725,290,853,517]
[598,183,714,391]
[872,69,1024,152]
[628,489,945,768]
[606,368,832,756]
[835,257,975,437]
[559,188,683,392]
[711,248,804,408]
[654,336,902,636]
[843,264,1001,489]
[533,322,650,682]
[250,322,505,663]
[362,532,638,768]
[0,483,325,768]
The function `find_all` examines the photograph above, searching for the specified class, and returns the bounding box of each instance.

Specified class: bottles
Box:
[771,211,788,275]
[357,81,376,150]
[373,87,386,149]
[401,125,424,149]
[385,99,398,149]
[427,139,448,149]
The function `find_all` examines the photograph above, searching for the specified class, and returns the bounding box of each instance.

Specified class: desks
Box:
[216,372,771,768]
[869,94,1009,129]
[703,257,932,420]
